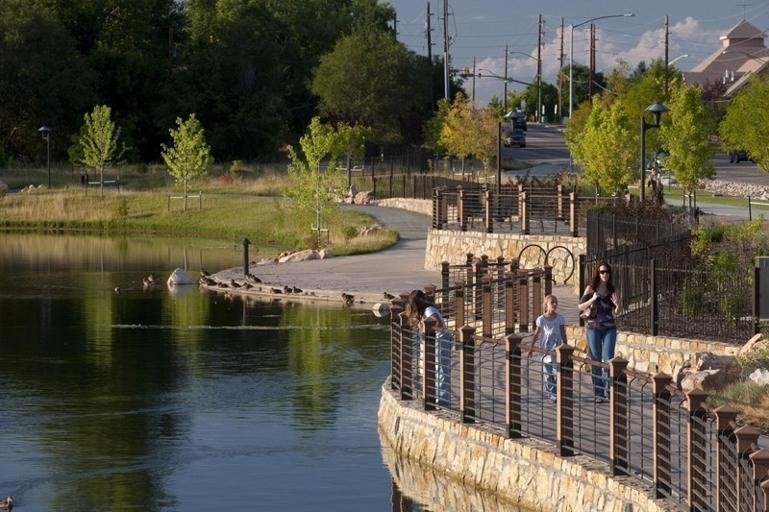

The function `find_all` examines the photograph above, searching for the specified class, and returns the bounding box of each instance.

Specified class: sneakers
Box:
[595,396,610,404]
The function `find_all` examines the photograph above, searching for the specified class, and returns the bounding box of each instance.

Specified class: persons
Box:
[403,302,422,397]
[527,295,569,404]
[407,289,454,410]
[576,260,624,405]
[647,167,662,202]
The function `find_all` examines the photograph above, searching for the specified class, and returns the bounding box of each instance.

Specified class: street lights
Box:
[38,125,51,190]
[478,68,508,117]
[667,54,689,67]
[569,13,635,120]
[509,52,543,124]
[640,100,671,201]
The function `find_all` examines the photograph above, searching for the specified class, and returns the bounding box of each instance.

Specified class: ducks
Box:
[115,285,120,291]
[284,285,292,294]
[292,285,303,294]
[383,291,395,300]
[270,287,282,294]
[143,273,163,292]
[342,293,354,305]
[199,268,261,291]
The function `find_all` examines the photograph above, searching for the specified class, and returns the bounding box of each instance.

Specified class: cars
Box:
[728,149,756,164]
[651,146,672,169]
[504,109,529,150]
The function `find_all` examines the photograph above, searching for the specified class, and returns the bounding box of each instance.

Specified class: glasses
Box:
[599,270,610,275]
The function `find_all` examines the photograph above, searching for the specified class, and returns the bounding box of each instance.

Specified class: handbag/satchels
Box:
[580,287,598,321]
